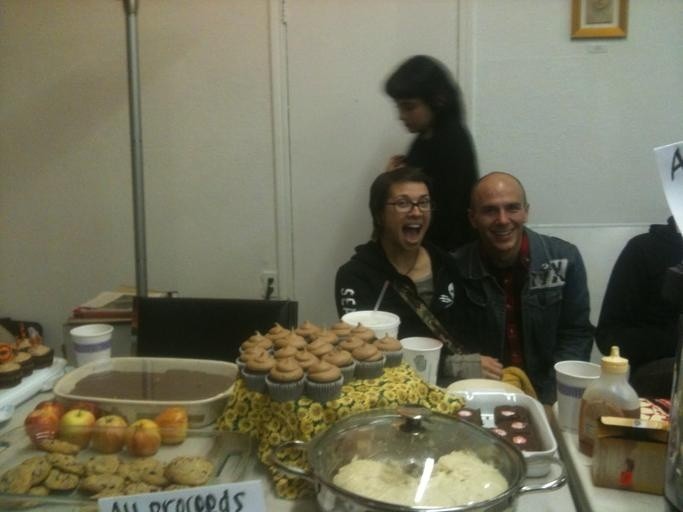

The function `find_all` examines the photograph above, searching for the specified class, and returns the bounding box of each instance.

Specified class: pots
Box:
[275,405,569,512]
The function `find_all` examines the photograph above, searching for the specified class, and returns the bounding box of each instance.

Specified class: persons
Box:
[380,54,481,251]
[596,217,683,410]
[452,173,595,406]
[334,168,505,385]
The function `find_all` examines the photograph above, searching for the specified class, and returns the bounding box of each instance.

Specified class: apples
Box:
[24,399,188,454]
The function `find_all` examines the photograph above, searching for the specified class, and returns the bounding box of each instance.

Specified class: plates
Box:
[446,378,557,476]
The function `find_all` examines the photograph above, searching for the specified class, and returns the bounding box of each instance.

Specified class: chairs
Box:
[127,294,299,362]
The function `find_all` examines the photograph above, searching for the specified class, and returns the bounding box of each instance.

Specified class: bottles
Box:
[578,345,640,457]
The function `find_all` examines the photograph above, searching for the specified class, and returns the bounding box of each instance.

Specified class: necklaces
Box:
[404,247,421,279]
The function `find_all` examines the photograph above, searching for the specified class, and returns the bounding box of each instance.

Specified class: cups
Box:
[401,337,443,385]
[554,360,600,432]
[69,323,114,366]
[344,311,399,341]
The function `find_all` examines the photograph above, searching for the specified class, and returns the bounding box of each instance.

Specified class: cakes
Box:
[0,340,54,387]
[236,317,404,403]
[456,401,541,453]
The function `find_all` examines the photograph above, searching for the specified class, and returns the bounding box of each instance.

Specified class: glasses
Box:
[385,197,434,213]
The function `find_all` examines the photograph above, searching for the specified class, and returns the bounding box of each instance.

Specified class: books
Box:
[64,316,134,326]
[69,288,180,320]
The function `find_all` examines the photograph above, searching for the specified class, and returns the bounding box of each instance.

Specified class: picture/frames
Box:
[569,0,629,40]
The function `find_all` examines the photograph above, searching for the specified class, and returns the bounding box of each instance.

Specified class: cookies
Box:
[1,438,213,506]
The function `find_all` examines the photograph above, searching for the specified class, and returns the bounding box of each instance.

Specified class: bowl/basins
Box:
[55,357,238,430]
